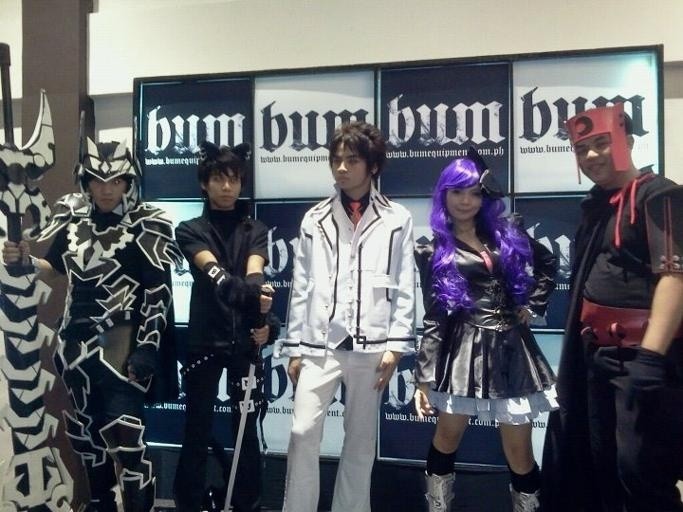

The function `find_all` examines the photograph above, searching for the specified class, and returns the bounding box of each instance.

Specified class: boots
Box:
[424,469,456,512]
[508,482,541,511]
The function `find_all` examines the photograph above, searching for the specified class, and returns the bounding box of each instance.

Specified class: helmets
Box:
[73,137,140,214]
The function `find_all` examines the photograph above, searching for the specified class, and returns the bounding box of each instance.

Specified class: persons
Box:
[277,120,416,511]
[172,137,283,510]
[1,141,183,511]
[534,100,683,511]
[409,153,563,512]
